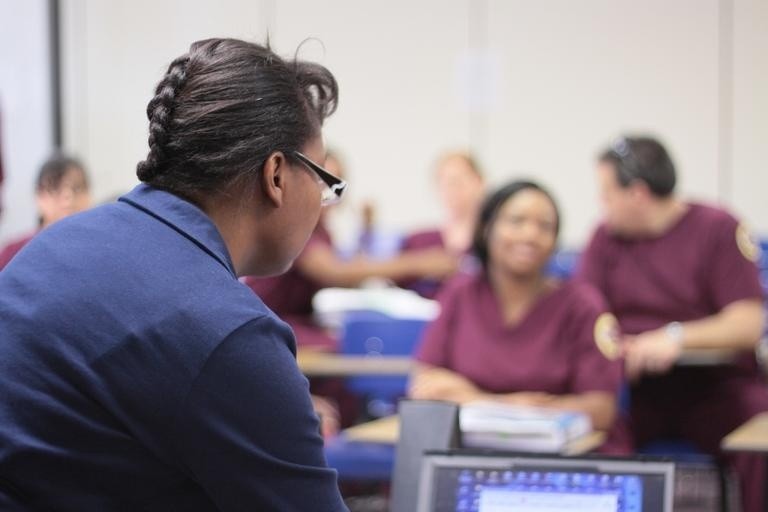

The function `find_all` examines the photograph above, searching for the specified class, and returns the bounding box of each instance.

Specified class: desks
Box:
[346,412,606,458]
[721,410,767,450]
[296,355,413,375]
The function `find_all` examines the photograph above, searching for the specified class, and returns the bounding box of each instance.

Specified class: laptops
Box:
[415,447,676,512]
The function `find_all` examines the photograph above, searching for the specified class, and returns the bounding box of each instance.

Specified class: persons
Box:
[1,155,90,269]
[0,37,349,512]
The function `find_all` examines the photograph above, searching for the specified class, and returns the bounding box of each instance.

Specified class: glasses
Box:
[284,148,348,207]
